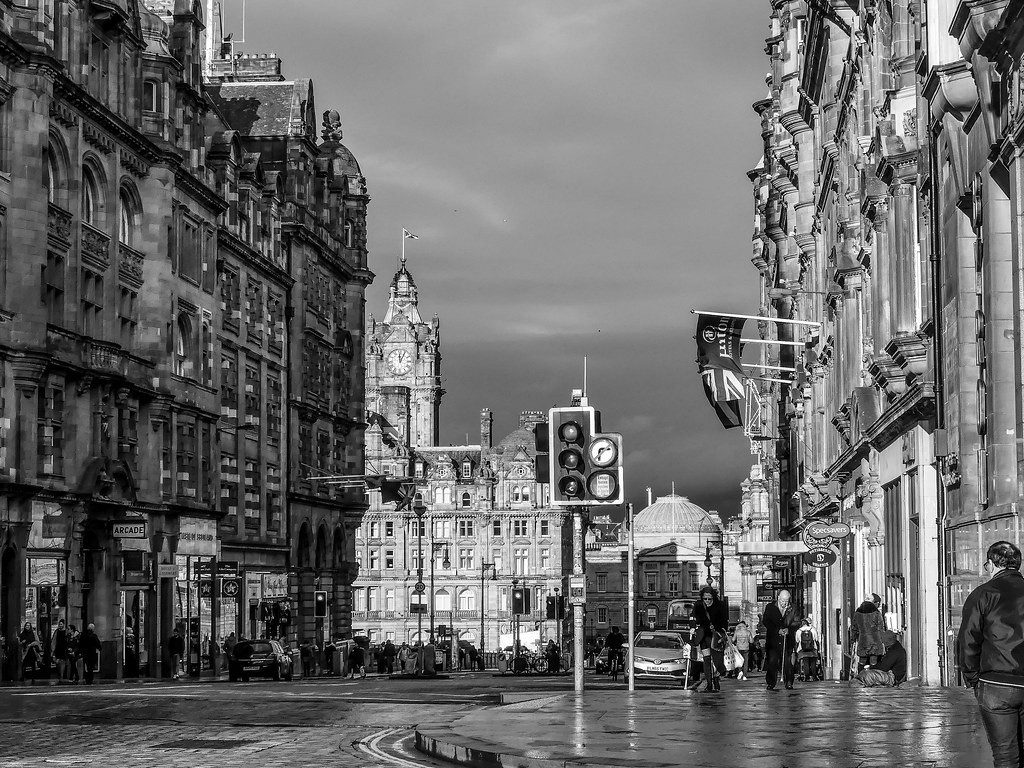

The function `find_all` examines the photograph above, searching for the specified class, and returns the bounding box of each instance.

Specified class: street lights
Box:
[480,557,497,669]
[429,534,451,646]
[704,538,714,587]
[414,504,427,645]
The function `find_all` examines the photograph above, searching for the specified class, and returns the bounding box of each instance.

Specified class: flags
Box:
[701,343,746,406]
[366,475,402,504]
[697,314,748,374]
[702,376,744,429]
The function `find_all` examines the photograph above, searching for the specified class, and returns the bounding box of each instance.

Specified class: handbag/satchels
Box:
[711,630,723,651]
[722,628,744,671]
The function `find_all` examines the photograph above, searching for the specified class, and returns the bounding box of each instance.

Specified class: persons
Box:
[195,636,220,672]
[849,630,907,687]
[301,639,313,675]
[374,639,395,674]
[324,641,337,663]
[49,619,102,685]
[397,641,424,673]
[223,632,240,681]
[850,593,887,675]
[446,646,452,671]
[497,650,506,661]
[124,626,135,676]
[457,646,465,671]
[732,590,819,689]
[238,634,290,655]
[546,639,560,673]
[469,645,478,671]
[956,540,1024,768]
[17,622,39,680]
[425,638,437,675]
[169,628,185,679]
[350,642,365,679]
[605,586,729,692]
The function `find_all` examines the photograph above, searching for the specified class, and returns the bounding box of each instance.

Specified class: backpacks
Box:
[798,627,816,652]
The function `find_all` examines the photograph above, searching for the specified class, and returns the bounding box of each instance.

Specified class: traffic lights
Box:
[511,589,524,614]
[314,591,327,618]
[546,597,555,619]
[549,407,625,505]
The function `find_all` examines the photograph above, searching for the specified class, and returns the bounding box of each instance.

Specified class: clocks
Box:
[387,348,413,376]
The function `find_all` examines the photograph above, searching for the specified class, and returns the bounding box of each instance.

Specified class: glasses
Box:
[984,562,998,571]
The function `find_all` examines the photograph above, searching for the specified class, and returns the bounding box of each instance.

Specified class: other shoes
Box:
[743,676,751,681]
[705,685,712,692]
[737,671,744,679]
[713,679,720,691]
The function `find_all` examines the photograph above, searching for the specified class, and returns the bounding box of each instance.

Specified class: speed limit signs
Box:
[415,582,426,592]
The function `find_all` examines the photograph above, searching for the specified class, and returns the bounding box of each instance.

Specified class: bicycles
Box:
[542,651,570,673]
[509,650,548,674]
[606,647,624,681]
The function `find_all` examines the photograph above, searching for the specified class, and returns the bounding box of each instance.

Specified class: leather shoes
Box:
[785,681,793,689]
[767,684,776,689]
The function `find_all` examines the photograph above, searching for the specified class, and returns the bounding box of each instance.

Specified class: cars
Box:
[229,639,293,682]
[656,628,700,681]
[620,632,696,686]
[594,647,626,674]
